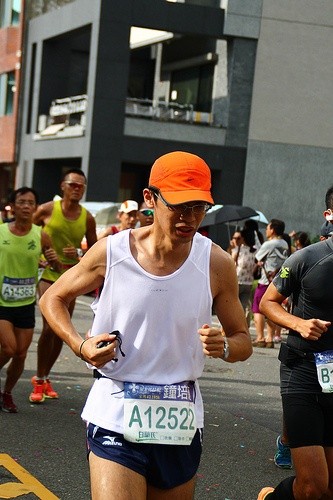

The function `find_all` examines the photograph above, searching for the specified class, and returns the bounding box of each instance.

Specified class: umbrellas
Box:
[225,210,269,232]
[199,204,259,242]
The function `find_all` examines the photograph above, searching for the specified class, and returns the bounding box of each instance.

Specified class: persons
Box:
[197,219,333,349]
[37,152,253,500]
[0,169,154,413]
[256,185,333,500]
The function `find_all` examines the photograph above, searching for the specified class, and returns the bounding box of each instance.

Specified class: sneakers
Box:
[28,379,46,403]
[1,391,16,413]
[31,375,58,399]
[273,436,293,470]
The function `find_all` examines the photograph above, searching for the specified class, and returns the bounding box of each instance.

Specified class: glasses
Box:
[14,200,36,206]
[152,191,213,215]
[64,181,86,189]
[139,209,154,216]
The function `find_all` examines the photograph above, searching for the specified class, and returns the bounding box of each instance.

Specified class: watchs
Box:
[76,247,84,257]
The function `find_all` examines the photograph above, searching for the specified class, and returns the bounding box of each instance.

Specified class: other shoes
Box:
[251,339,266,348]
[273,336,282,342]
[257,486,275,500]
[264,341,274,349]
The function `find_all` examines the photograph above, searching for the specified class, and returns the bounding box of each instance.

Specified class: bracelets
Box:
[80,339,88,360]
[216,336,230,361]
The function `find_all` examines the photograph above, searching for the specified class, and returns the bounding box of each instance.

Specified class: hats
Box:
[118,200,139,214]
[148,151,216,206]
